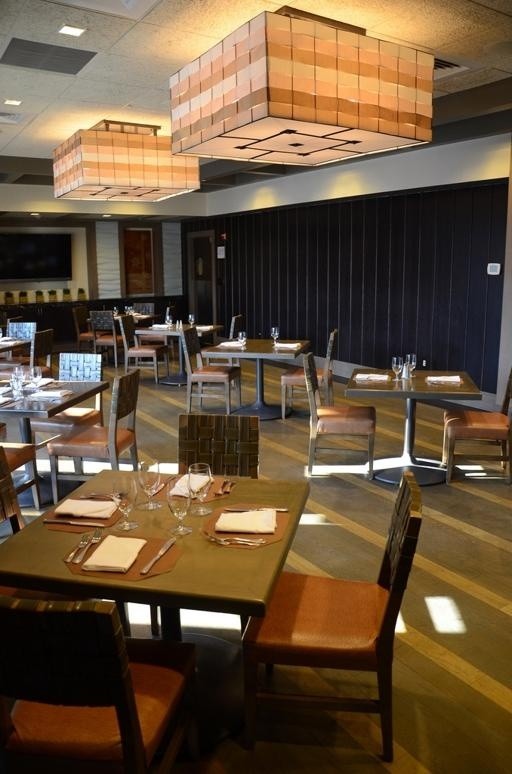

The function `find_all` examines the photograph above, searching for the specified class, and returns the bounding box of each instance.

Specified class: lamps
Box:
[168,5,437,168]
[50,120,200,202]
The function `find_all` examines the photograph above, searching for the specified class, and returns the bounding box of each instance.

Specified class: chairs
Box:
[0,596,196,768]
[88,310,128,368]
[0,448,25,603]
[46,370,140,507]
[21,353,104,445]
[2,328,54,378]
[118,316,170,384]
[178,326,242,416]
[1,439,42,511]
[301,351,376,480]
[442,367,511,485]
[281,328,340,422]
[199,314,244,386]
[0,315,24,358]
[2,322,37,366]
[242,470,427,759]
[73,305,109,361]
[179,412,260,480]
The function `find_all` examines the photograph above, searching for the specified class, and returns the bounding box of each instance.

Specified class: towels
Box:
[167,474,213,500]
[216,509,276,533]
[53,499,120,518]
[82,534,147,573]
[426,375,462,383]
[355,374,389,381]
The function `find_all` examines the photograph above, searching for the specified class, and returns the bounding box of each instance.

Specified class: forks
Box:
[224,479,237,495]
[216,476,230,496]
[73,526,104,564]
[62,532,91,562]
[199,526,266,548]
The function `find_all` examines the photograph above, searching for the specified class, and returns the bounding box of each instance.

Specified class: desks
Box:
[135,324,208,386]
[1,340,31,373]
[201,335,304,421]
[1,381,113,508]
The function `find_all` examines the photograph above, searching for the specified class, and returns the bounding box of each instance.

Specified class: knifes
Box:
[41,516,106,527]
[141,536,176,573]
[150,474,177,495]
[223,506,289,513]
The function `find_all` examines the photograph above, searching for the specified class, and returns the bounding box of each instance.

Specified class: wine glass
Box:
[7,362,44,397]
[188,314,196,329]
[187,462,213,516]
[166,471,194,536]
[136,459,164,512]
[238,330,248,349]
[112,476,140,531]
[166,315,175,330]
[392,381,402,390]
[391,356,404,382]
[405,353,417,379]
[270,327,281,347]
[10,394,51,412]
[112,305,133,315]
[402,377,415,391]
